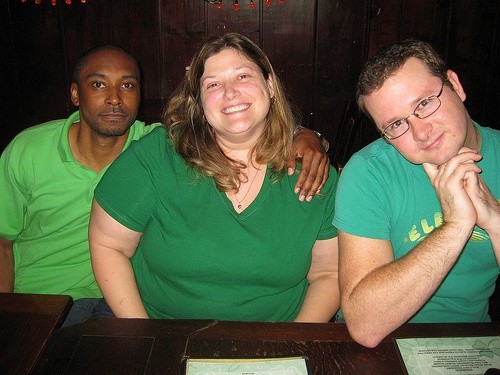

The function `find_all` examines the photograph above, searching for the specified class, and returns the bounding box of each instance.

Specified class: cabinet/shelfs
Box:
[0,0,500,171]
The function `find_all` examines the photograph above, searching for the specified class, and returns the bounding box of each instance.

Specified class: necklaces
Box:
[235,164,261,209]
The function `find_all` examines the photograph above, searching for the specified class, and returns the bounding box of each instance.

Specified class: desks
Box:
[0,292,500,375]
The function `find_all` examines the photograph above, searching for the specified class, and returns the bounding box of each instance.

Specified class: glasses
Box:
[379,83,444,140]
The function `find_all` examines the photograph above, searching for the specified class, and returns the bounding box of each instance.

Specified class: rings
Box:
[313,160,320,164]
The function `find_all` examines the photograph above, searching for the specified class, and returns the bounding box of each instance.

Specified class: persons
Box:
[0,43,330,327]
[331,41,500,349]
[88,33,341,323]
